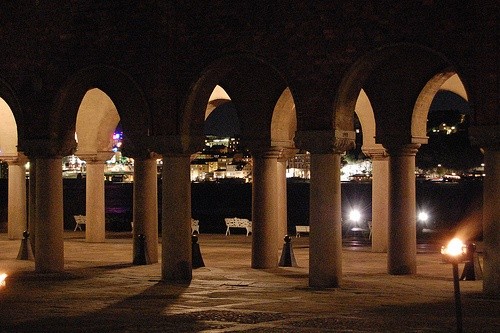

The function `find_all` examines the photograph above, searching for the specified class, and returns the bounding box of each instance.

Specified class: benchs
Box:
[224,217,252,235]
[72,214,87,231]
[295,224,311,237]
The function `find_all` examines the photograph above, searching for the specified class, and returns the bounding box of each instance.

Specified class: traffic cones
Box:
[459,243,484,281]
[16,231,35,261]
[190,235,205,270]
[278,234,297,267]
[134,234,150,264]
